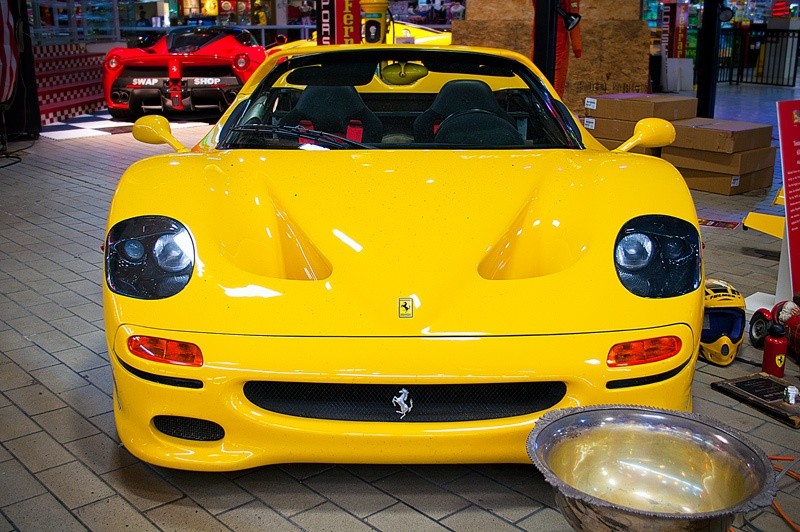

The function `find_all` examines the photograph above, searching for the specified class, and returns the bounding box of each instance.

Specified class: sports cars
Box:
[265,18,452,57]
[104,42,705,471]
[104,26,288,119]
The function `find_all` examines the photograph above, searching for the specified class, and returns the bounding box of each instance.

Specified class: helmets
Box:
[697,279,747,367]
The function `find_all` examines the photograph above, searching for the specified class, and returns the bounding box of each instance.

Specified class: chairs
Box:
[278,84,383,145]
[411,79,511,146]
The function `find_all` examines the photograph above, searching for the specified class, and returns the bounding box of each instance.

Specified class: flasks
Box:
[763,323,788,377]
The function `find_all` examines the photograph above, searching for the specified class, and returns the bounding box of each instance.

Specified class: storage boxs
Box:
[584,93,700,120]
[670,116,774,155]
[659,143,778,174]
[582,114,640,138]
[596,137,660,158]
[675,165,775,196]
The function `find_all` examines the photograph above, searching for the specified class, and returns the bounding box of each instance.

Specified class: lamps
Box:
[557,5,582,31]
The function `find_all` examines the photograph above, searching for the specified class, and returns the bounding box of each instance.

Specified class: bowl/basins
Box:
[526,404,778,532]
